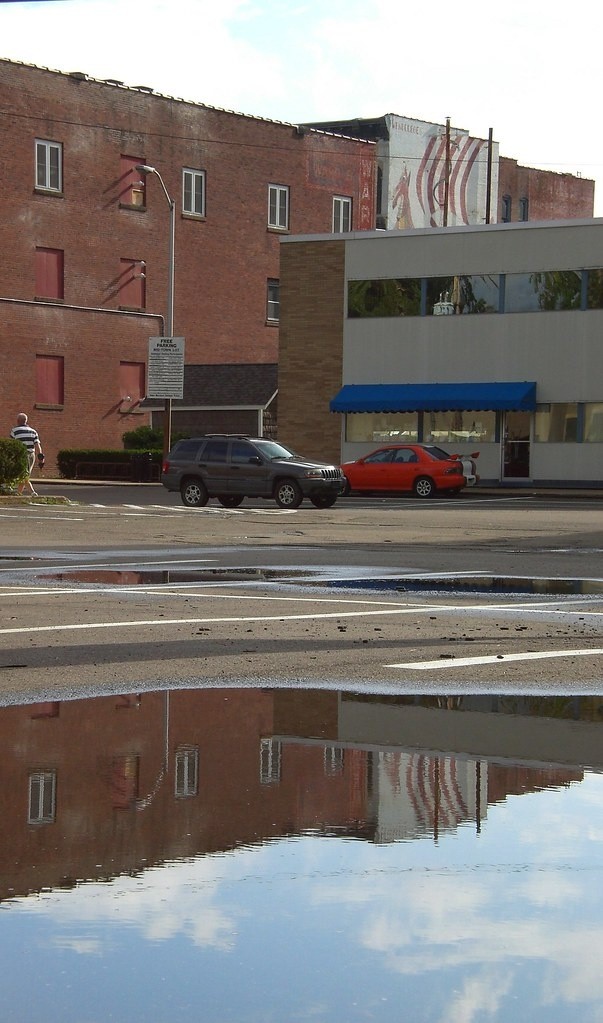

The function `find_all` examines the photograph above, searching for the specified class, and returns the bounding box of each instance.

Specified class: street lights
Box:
[135,164,176,457]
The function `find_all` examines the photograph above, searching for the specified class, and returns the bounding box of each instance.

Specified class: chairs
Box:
[396,457,403,462]
[408,455,417,462]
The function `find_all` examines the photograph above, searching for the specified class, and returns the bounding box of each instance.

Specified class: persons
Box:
[9,413,45,496]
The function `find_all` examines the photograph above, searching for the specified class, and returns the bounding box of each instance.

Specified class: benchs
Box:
[76,462,132,480]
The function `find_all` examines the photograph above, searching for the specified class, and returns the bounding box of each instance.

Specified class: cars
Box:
[335,444,480,499]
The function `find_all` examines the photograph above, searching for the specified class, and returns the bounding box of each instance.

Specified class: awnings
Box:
[329,382,536,414]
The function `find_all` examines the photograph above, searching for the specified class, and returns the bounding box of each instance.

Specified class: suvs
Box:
[160,433,347,508]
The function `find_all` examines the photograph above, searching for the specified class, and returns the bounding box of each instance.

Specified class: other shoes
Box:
[30,492,38,497]
